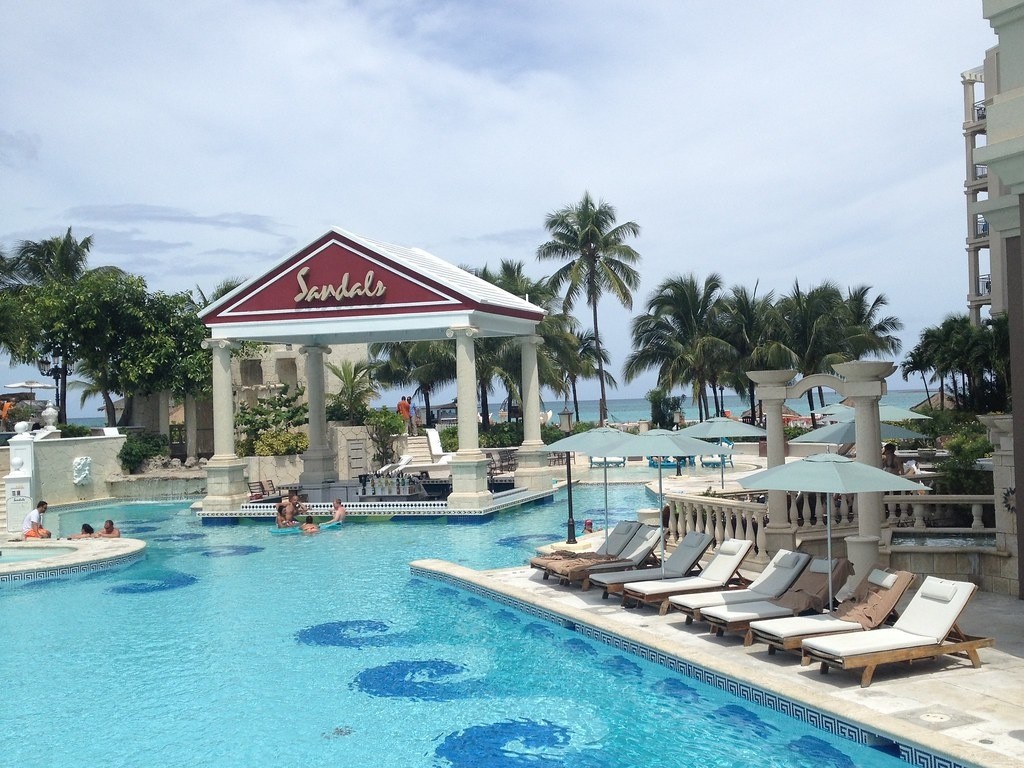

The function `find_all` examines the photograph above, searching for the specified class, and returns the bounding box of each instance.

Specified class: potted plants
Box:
[976,411,1014,450]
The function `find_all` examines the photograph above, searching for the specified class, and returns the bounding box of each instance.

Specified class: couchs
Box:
[646,456,696,468]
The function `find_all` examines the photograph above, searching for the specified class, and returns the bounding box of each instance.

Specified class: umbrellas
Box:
[736,453,933,613]
[5,380,59,415]
[671,416,767,489]
[786,402,933,452]
[579,424,737,579]
[535,424,641,554]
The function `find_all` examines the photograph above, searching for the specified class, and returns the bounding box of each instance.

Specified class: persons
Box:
[652,456,690,468]
[581,519,603,534]
[882,443,904,476]
[397,396,418,437]
[23,501,51,539]
[70,520,120,539]
[276,496,346,532]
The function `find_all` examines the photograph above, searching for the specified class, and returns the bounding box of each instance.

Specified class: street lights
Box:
[557,406,577,544]
[38,353,75,407]
[674,411,682,477]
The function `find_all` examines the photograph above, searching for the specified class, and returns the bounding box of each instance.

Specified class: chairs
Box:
[697,556,855,648]
[531,520,643,586]
[748,562,916,668]
[668,548,812,636]
[545,524,672,586]
[486,449,518,476]
[621,538,753,617]
[247,480,276,497]
[800,576,995,688]
[547,450,575,467]
[588,531,715,608]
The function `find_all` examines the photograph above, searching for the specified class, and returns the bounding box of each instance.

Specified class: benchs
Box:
[590,456,627,469]
[700,437,735,469]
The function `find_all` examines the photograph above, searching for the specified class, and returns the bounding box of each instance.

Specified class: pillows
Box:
[921,581,958,601]
[615,523,632,535]
[773,553,800,569]
[638,526,656,540]
[719,540,742,555]
[684,533,704,547]
[810,558,838,574]
[867,568,898,590]
